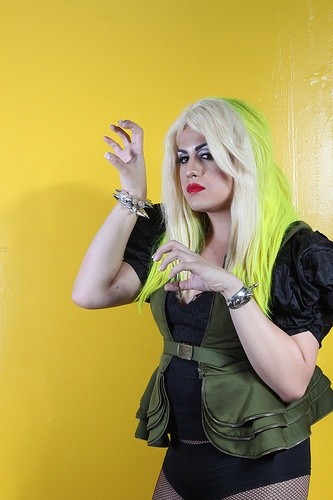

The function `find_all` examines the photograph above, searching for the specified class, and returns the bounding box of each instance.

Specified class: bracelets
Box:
[113,188,155,220]
[224,282,261,311]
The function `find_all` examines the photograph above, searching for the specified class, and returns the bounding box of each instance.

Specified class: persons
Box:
[74,94,332,499]
